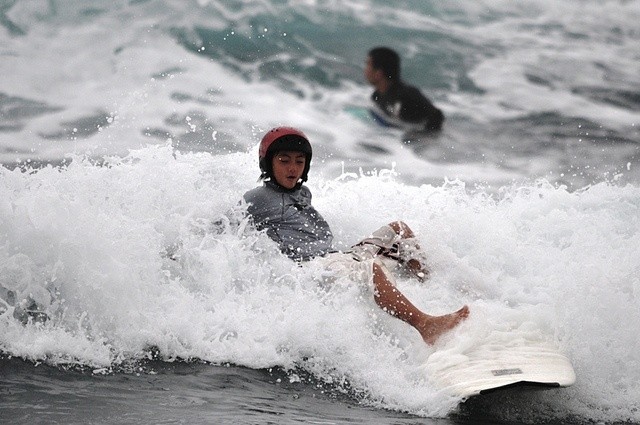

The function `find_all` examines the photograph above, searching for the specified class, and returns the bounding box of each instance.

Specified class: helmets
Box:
[259,126,313,181]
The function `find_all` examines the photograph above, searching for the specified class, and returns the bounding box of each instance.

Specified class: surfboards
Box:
[433,342,577,403]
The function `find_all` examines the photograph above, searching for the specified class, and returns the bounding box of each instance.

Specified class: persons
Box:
[363,45,444,137]
[212,127,471,346]
